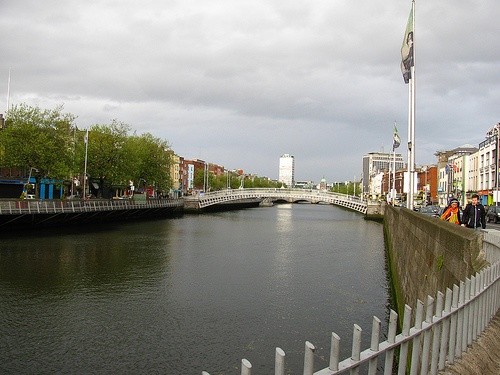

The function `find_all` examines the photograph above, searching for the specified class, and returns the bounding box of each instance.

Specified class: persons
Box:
[389,198,394,206]
[441,199,461,225]
[461,194,486,229]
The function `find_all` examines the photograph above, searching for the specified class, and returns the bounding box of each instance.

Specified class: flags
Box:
[393,123,400,151]
[401,3,415,70]
[401,61,411,84]
[84,131,88,145]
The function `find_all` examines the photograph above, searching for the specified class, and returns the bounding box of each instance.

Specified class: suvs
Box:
[486,206,500,224]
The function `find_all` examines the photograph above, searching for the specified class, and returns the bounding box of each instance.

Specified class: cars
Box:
[113,195,132,200]
[357,192,470,224]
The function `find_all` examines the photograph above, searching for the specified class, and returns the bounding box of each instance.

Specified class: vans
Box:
[23,195,42,200]
